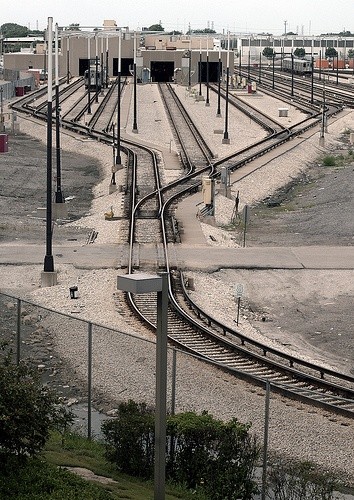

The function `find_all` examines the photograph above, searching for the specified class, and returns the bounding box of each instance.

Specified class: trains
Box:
[85,66,105,93]
[283,57,312,76]
